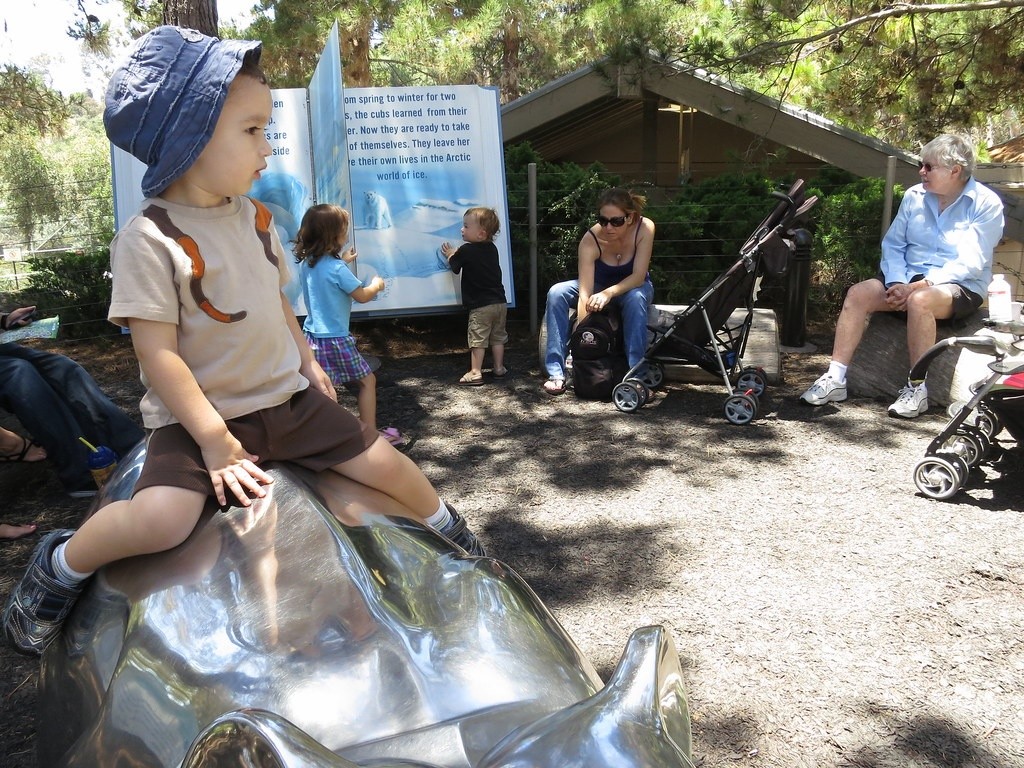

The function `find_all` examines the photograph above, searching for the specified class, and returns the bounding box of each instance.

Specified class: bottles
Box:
[988,273,1012,321]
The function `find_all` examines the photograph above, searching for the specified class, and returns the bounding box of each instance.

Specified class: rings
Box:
[596,302,599,305]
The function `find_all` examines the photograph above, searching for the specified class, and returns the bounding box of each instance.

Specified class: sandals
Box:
[493,366,508,379]
[458,372,485,386]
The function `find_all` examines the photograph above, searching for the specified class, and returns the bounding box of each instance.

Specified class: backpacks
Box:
[570,305,632,402]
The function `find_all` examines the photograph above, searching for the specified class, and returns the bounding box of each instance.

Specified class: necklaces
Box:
[608,240,627,260]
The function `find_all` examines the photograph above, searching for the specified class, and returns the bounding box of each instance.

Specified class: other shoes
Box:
[377,430,403,445]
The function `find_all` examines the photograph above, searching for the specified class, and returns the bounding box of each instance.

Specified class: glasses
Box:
[598,214,630,227]
[918,161,933,171]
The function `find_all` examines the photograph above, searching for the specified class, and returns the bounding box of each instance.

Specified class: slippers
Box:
[0,434,50,463]
[0,522,35,542]
[636,385,656,402]
[543,373,571,395]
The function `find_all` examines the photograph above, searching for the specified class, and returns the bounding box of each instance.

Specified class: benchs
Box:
[839,301,1023,408]
[538,302,783,384]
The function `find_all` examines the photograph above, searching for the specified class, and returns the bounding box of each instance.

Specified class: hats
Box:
[102,24,262,199]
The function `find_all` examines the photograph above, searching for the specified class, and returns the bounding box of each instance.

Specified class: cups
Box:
[1011,302,1024,321]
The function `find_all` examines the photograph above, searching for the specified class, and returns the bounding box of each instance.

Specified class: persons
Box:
[287,202,403,445]
[800,134,1005,419]
[440,207,509,386]
[0,308,147,543]
[543,188,657,402]
[4,26,487,658]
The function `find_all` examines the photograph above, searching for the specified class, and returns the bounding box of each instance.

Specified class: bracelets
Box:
[926,280,934,286]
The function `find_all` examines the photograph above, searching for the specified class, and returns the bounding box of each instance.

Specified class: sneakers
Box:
[887,379,929,418]
[440,500,487,558]
[1,528,95,660]
[799,371,848,407]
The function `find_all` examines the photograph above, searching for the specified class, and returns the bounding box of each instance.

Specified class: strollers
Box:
[611,177,813,424]
[914,344,1022,502]
